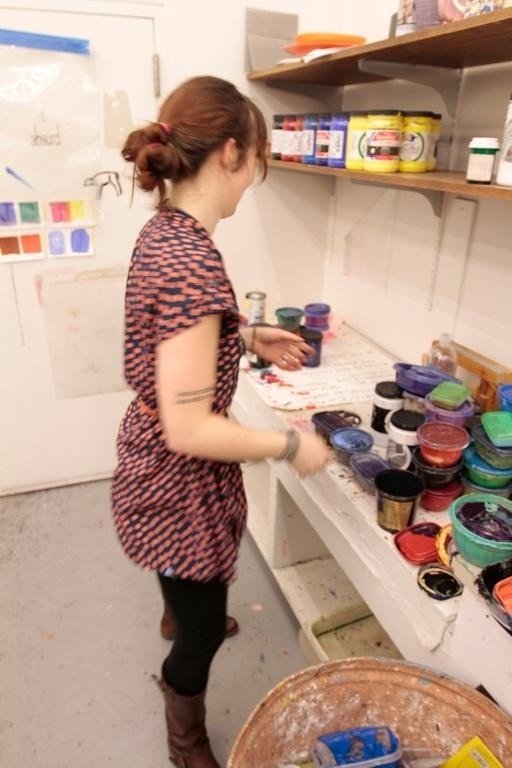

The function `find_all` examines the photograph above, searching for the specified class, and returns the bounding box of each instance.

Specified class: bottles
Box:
[429,332,457,378]
[465,93,512,187]
[271,112,345,169]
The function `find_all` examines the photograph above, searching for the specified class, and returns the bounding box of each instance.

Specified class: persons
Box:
[111,72,330,768]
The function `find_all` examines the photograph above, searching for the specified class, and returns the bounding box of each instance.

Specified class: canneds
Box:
[273,110,443,173]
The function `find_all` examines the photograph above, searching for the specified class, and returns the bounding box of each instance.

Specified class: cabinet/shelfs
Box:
[226,313,512,718]
[249,7,512,202]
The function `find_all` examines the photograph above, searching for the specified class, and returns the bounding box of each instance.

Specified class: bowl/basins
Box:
[449,493,512,569]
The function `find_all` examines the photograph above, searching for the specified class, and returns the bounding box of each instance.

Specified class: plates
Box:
[284,31,366,55]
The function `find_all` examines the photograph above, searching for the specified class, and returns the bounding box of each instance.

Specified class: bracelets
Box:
[249,323,259,352]
[281,425,303,461]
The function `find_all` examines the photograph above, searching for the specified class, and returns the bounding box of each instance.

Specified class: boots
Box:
[158,655,221,768]
[156,571,239,642]
